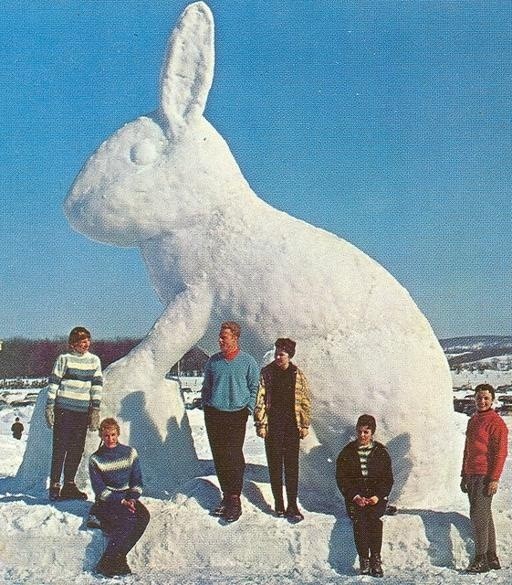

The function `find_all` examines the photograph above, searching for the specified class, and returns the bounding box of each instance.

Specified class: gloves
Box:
[86,407,100,432]
[44,402,54,429]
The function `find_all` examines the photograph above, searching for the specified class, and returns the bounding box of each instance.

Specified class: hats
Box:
[275,338,296,356]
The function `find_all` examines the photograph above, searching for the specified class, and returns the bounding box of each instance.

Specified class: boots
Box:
[95,552,131,577]
[360,552,383,577]
[459,552,501,575]
[48,482,87,501]
[275,495,304,521]
[209,492,243,522]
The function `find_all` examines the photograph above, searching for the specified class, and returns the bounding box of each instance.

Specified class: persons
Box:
[88,418,151,579]
[459,383,510,574]
[200,321,312,523]
[335,414,394,578]
[44,327,104,501]
[11,417,24,440]
